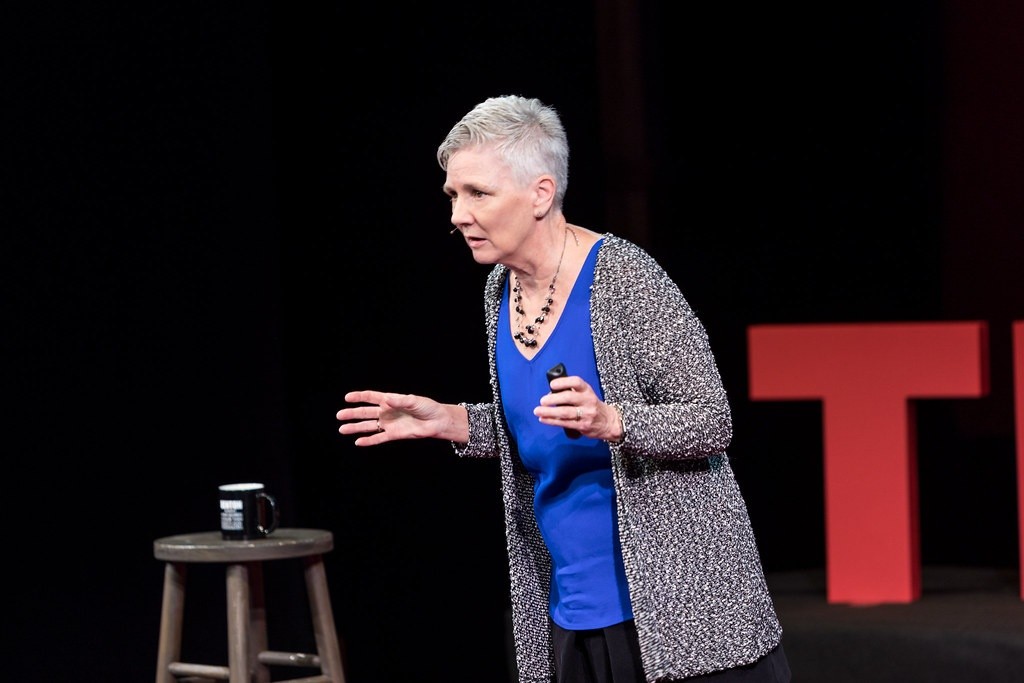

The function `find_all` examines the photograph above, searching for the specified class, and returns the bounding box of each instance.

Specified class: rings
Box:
[376,419,381,433]
[577,405,583,421]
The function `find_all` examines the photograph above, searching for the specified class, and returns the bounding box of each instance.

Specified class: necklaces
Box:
[509,227,578,346]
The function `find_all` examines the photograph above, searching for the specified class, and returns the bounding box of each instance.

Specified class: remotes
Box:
[546,362,582,439]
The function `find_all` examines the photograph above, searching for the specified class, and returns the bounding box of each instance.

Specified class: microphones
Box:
[450,228,457,234]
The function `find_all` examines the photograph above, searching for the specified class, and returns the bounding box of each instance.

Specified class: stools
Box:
[154,527,348,683]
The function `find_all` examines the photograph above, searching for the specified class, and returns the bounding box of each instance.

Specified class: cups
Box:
[218,482,277,539]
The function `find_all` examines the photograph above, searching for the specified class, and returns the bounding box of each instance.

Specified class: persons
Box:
[335,95,794,683]
